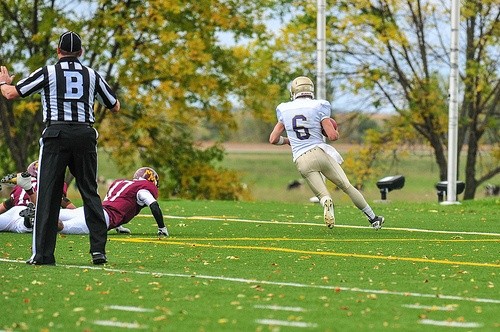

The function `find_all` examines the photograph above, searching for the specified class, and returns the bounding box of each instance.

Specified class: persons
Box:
[1,166,169,236]
[268,76,385,231]
[0,161,75,234]
[0,31,120,265]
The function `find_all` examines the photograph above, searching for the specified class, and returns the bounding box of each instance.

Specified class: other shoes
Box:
[369,215,385,231]
[323,198,335,229]
[18,202,36,228]
[92,251,108,264]
[1,171,32,188]
[25,258,56,265]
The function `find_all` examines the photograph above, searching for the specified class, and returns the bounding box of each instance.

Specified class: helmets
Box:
[26,160,38,177]
[133,167,159,190]
[289,76,314,101]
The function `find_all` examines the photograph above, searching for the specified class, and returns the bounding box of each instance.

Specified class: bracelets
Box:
[0,81,7,86]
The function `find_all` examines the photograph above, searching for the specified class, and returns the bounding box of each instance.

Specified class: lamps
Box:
[436,182,465,202]
[376,175,405,200]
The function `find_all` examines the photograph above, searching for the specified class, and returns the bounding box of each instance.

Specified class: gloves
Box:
[158,227,168,236]
[116,226,131,234]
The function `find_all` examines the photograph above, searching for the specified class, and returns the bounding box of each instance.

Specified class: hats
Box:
[59,30,81,52]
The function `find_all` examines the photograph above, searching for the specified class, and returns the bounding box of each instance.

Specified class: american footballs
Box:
[322,118,337,137]
